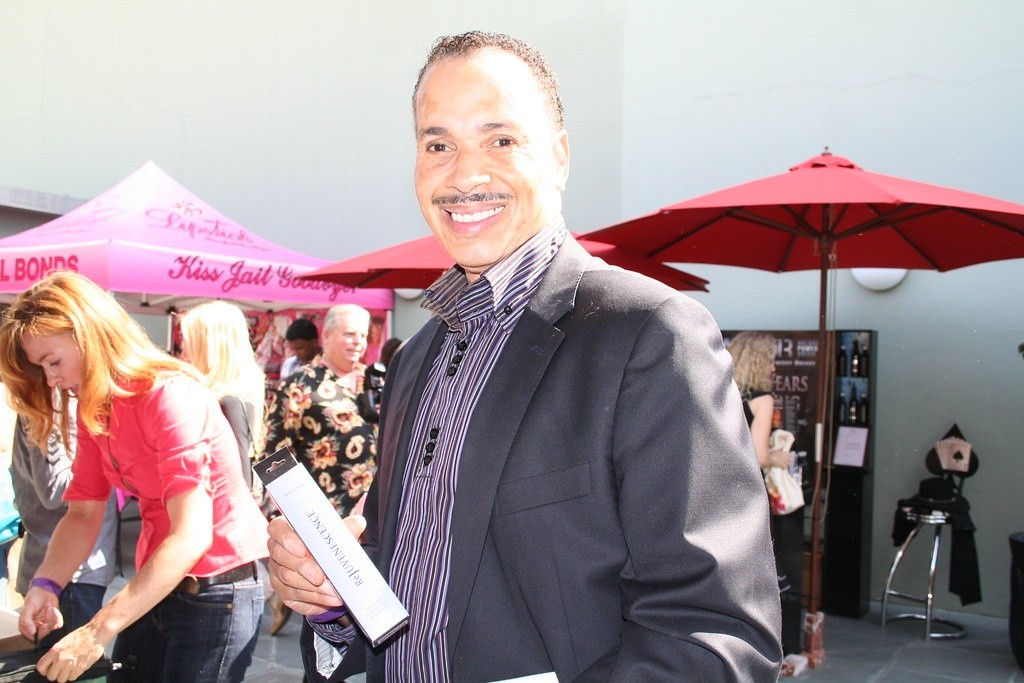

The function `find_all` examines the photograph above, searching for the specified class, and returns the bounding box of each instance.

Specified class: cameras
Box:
[356,362,385,423]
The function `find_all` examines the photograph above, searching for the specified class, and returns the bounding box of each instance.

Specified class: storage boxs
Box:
[252,445,410,648]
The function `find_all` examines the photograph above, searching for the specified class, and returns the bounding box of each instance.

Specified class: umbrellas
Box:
[577,149,1024,666]
[296,232,719,295]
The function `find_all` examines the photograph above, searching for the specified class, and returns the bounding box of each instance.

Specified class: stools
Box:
[880,424,980,640]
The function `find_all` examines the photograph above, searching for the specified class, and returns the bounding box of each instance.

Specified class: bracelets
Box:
[30,577,63,597]
[305,607,347,623]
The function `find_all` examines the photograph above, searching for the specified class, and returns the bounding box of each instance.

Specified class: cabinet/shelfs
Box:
[720,329,878,620]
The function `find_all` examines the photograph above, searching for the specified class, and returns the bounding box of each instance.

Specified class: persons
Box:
[253,31,760,683]
[0,301,405,683]
[0,271,269,683]
[725,331,793,598]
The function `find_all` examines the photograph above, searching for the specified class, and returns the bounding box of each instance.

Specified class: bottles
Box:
[852,341,860,376]
[839,345,847,376]
[849,387,858,425]
[861,394,867,426]
[861,347,868,376]
[838,393,846,424]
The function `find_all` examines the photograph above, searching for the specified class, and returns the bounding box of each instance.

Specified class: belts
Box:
[181,560,256,595]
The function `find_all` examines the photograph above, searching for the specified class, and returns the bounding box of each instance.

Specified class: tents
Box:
[0,159,394,347]
